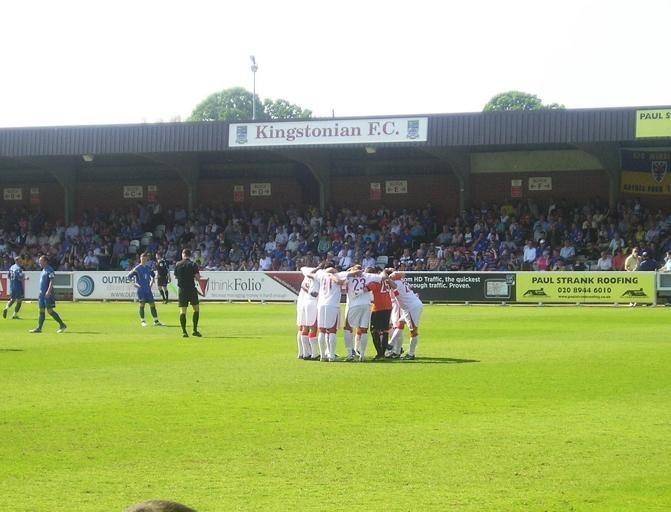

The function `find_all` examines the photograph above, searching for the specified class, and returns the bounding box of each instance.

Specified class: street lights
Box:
[250,56,259,121]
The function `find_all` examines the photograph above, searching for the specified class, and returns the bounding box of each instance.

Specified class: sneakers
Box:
[297,347,415,362]
[140,320,164,327]
[181,331,202,337]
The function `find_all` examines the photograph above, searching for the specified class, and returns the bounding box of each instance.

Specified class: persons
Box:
[29,255,66,333]
[174,249,202,337]
[127,253,164,327]
[3,256,25,319]
[154,251,171,304]
[1,196,671,271]
[297,259,423,362]
